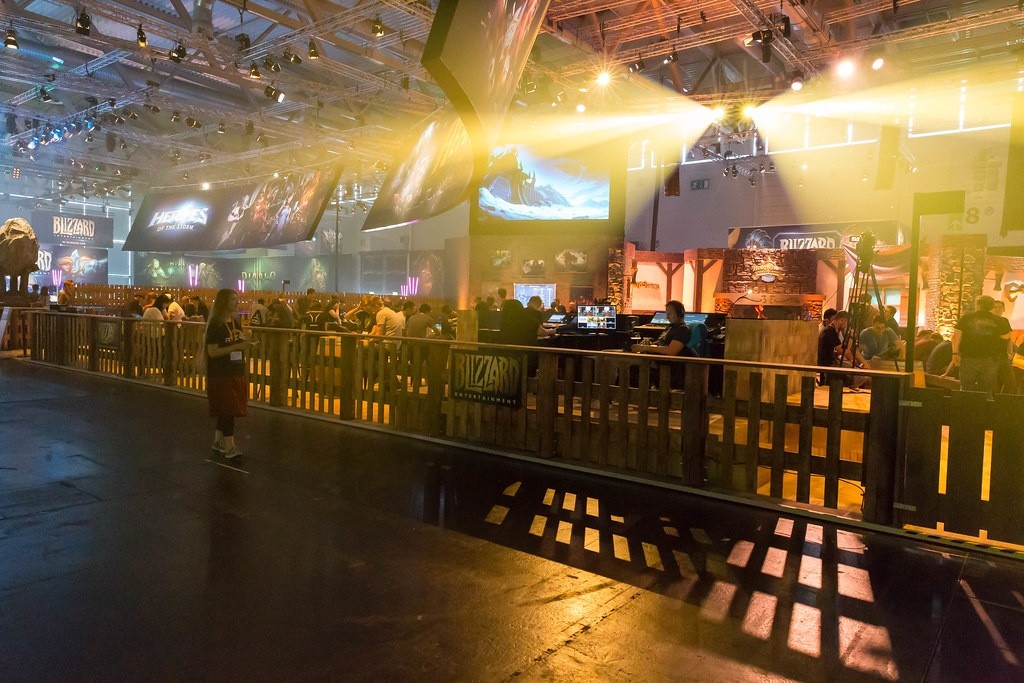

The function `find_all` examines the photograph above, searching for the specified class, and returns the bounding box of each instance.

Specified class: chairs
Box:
[632,322,708,387]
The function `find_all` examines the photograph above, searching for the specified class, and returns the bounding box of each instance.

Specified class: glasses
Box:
[232,298,240,303]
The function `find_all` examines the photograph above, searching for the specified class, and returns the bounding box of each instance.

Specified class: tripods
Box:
[840,254,901,389]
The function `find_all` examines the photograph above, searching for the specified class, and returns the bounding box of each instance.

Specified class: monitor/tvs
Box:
[514,282,557,311]
[577,305,616,333]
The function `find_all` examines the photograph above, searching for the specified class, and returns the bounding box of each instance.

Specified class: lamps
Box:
[263,58,282,74]
[249,60,261,78]
[76,9,90,37]
[627,61,645,74]
[371,15,388,37]
[136,25,147,48]
[169,45,186,63]
[3,22,19,49]
[691,136,774,188]
[263,82,286,104]
[308,38,319,59]
[665,52,678,64]
[13,74,228,210]
[246,119,269,149]
[281,49,303,65]
[791,77,804,92]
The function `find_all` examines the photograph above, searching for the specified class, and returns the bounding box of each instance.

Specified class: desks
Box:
[633,324,666,339]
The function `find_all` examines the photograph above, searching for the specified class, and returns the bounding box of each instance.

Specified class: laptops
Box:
[431,323,442,332]
[547,313,566,323]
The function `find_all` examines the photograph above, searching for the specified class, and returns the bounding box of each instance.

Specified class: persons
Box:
[251,288,458,388]
[124,295,208,340]
[204,288,258,458]
[473,288,575,342]
[631,301,690,354]
[913,295,1015,396]
[29,281,77,309]
[819,294,901,393]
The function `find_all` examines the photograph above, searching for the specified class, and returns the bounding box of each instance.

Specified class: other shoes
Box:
[212,440,242,458]
